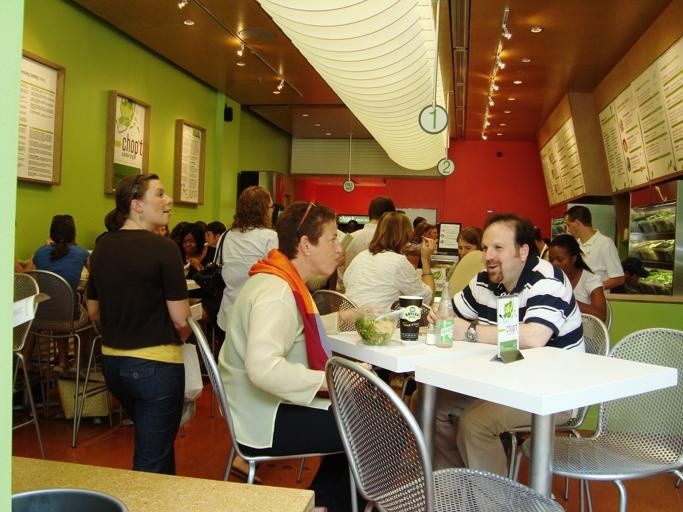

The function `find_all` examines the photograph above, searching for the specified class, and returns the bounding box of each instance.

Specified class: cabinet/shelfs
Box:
[629,182,682,294]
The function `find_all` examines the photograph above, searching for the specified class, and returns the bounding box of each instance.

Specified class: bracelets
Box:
[420,269,435,277]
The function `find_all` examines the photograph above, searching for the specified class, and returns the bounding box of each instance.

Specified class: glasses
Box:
[296,201,318,233]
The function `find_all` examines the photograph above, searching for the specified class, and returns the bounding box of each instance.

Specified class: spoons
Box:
[373,308,407,323]
[184,260,190,270]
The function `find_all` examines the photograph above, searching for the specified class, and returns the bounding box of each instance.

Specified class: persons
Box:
[425,209,587,511]
[86,171,192,478]
[549,235,609,324]
[176,222,217,281]
[429,223,439,241]
[411,216,427,229]
[204,219,228,247]
[149,223,170,239]
[413,222,433,246]
[610,257,651,296]
[343,218,360,235]
[217,200,412,512]
[211,185,279,351]
[337,196,399,296]
[564,205,624,294]
[446,226,483,284]
[194,220,208,233]
[169,220,189,241]
[341,210,437,322]
[443,250,491,300]
[31,215,93,378]
[15,255,37,276]
[528,224,554,263]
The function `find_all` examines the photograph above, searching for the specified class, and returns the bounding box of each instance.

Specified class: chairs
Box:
[12,273,46,460]
[313,290,362,332]
[514,328,681,511]
[324,357,565,512]
[74,319,116,439]
[391,303,433,326]
[29,270,113,449]
[448,313,609,482]
[185,315,359,510]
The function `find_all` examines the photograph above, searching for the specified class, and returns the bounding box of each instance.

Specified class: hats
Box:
[622,257,651,278]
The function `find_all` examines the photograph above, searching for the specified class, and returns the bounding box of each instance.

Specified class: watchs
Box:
[465,319,480,341]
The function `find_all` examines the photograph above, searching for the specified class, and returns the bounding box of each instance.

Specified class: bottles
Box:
[435,282,454,348]
[426,290,442,344]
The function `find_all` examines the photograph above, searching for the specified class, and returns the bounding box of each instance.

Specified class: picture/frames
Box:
[17,49,65,185]
[174,119,206,205]
[104,91,152,193]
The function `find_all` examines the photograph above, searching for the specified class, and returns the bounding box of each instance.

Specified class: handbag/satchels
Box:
[198,229,232,320]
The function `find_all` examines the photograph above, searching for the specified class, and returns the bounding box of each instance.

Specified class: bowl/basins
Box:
[632,215,675,233]
[354,307,400,348]
[631,239,674,261]
[629,266,673,296]
[184,269,189,278]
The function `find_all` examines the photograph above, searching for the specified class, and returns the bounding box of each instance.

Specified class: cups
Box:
[398,295,425,343]
